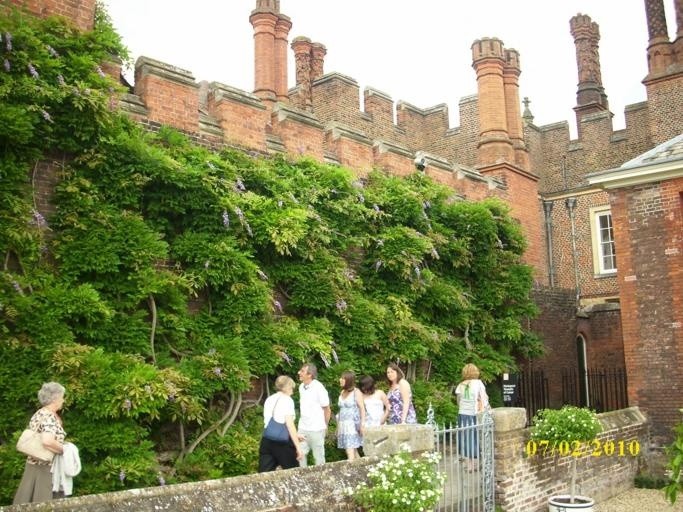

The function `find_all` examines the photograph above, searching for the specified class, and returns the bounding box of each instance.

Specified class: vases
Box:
[546,494,594,511]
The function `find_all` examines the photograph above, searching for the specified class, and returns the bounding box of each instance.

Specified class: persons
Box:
[383,362,417,424]
[11,380,80,503]
[333,370,366,461]
[296,362,331,468]
[357,372,390,428]
[257,374,306,472]
[454,363,489,470]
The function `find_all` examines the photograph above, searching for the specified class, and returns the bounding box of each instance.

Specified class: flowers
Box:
[341,442,446,511]
[528,402,604,502]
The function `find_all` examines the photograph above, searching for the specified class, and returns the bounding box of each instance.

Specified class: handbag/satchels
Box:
[264,418,290,442]
[475,392,491,414]
[16,429,55,462]
[354,415,361,431]
